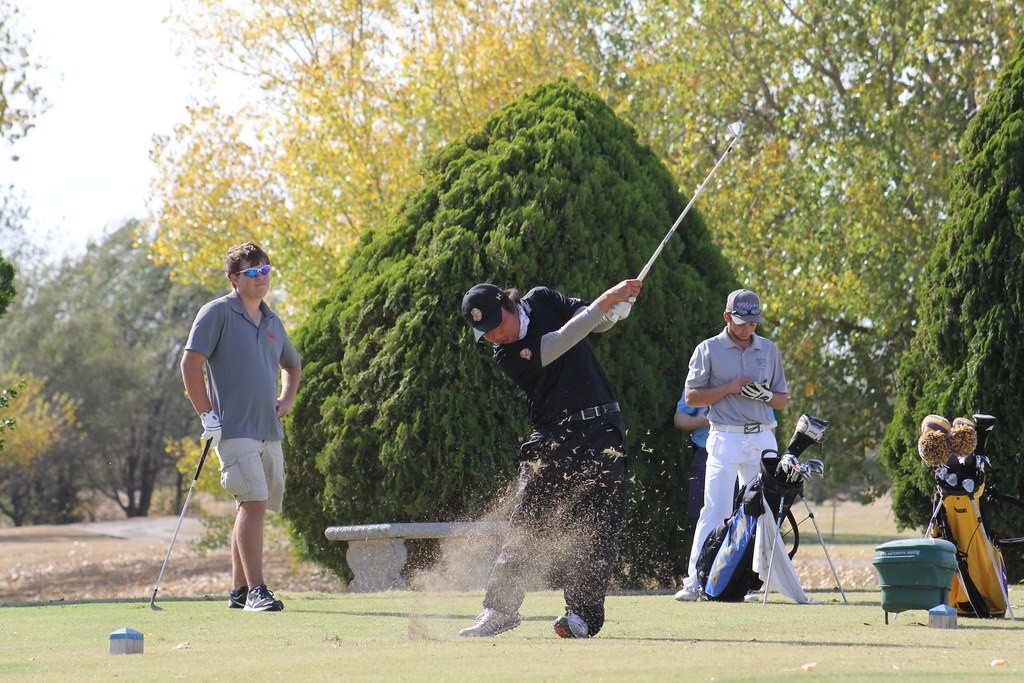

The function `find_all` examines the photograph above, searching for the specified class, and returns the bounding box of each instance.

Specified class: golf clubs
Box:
[150,437,213,610]
[976,455,991,475]
[775,453,824,484]
[628,120,745,303]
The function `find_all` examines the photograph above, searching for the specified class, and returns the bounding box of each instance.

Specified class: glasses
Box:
[234,264,271,277]
[727,307,762,317]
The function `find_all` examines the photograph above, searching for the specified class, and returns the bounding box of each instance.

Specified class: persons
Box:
[675,388,710,535]
[180,242,301,612]
[459,280,642,639]
[674,289,788,603]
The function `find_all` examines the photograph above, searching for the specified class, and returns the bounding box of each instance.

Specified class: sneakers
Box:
[460,607,521,639]
[228,589,246,608]
[675,586,699,602]
[243,584,284,610]
[555,610,588,637]
[745,590,758,601]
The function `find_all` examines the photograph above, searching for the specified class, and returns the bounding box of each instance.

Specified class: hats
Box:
[461,285,507,343]
[724,289,764,326]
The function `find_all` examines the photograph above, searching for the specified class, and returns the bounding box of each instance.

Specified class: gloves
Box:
[740,381,773,404]
[199,410,221,448]
[609,295,636,320]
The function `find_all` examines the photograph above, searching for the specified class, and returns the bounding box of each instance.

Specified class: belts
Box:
[562,401,620,422]
[710,420,778,434]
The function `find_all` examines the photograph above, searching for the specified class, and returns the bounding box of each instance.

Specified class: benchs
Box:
[323,521,511,593]
